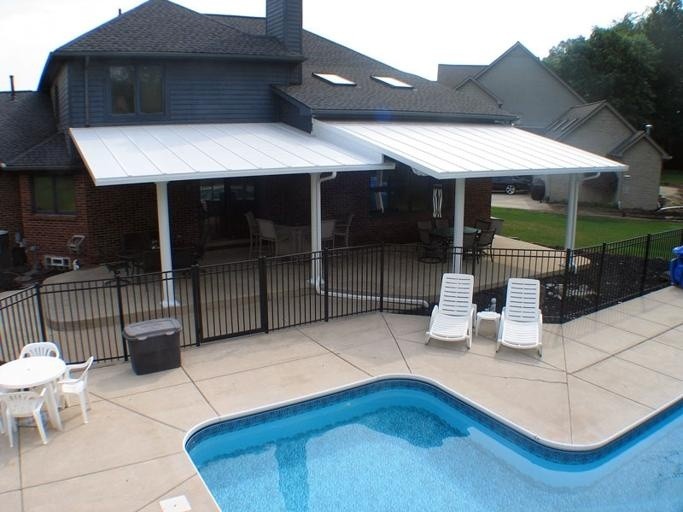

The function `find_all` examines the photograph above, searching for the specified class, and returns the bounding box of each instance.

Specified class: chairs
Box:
[0,342,93,447]
[424,272,477,349]
[118,230,204,279]
[415,217,496,264]
[495,277,543,357]
[206,200,226,225]
[244,210,354,267]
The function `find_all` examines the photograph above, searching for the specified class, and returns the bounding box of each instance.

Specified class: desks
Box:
[475,311,501,338]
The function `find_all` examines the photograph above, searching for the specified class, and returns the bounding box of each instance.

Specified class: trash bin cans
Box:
[669,246,683,287]
[121,318,181,375]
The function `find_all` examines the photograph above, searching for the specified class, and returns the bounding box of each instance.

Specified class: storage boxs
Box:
[122,318,182,375]
